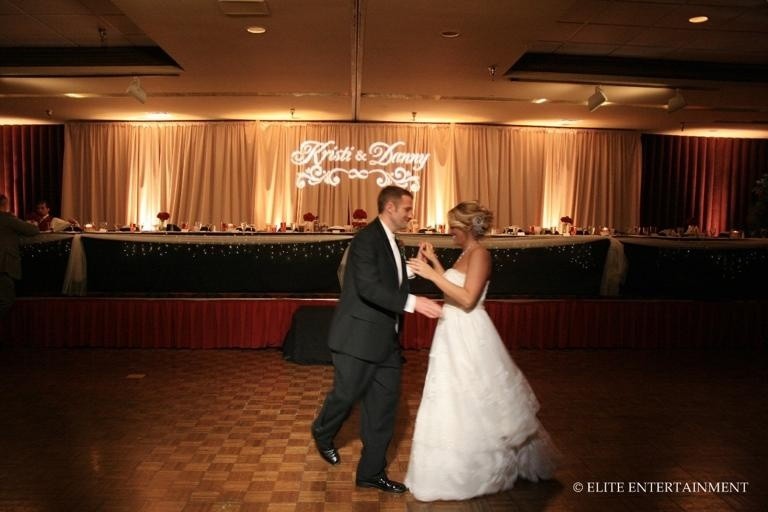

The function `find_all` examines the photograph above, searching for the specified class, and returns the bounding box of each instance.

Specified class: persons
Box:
[25,199,79,231]
[404,198,497,502]
[0,193,41,318]
[310,183,444,494]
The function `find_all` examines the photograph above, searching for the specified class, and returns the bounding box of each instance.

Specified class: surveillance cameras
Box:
[45,110,52,116]
[680,120,688,130]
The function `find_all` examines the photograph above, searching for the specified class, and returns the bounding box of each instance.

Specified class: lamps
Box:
[587,86,606,113]
[125,76,147,102]
[667,89,687,111]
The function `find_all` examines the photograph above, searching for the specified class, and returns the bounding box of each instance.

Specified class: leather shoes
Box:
[319,446,340,465]
[356,475,406,493]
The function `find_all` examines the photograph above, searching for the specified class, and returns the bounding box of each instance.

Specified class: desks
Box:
[0,233,768,353]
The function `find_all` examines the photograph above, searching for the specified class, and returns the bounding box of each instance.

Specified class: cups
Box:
[528,225,615,237]
[437,224,445,235]
[128,222,298,234]
